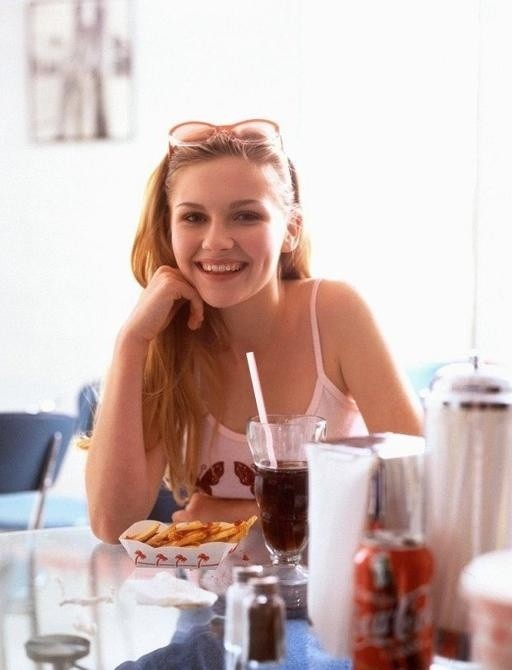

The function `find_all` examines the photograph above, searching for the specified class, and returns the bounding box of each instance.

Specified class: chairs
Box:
[75,381,101,440]
[0,406,84,533]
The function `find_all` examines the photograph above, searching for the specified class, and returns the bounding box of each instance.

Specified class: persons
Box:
[85,118,424,545]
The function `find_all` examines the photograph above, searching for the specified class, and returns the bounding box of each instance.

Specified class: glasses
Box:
[169,119,284,159]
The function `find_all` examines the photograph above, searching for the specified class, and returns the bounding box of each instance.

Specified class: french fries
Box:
[129,515,257,546]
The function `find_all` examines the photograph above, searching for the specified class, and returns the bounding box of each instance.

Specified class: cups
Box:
[304,429,425,656]
[242,417,330,586]
[456,548,512,670]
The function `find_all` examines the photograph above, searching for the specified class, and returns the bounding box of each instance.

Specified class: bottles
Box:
[422,356,511,644]
[223,568,264,654]
[245,576,287,667]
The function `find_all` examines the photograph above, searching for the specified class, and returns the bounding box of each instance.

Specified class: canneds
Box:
[350,531,434,669]
